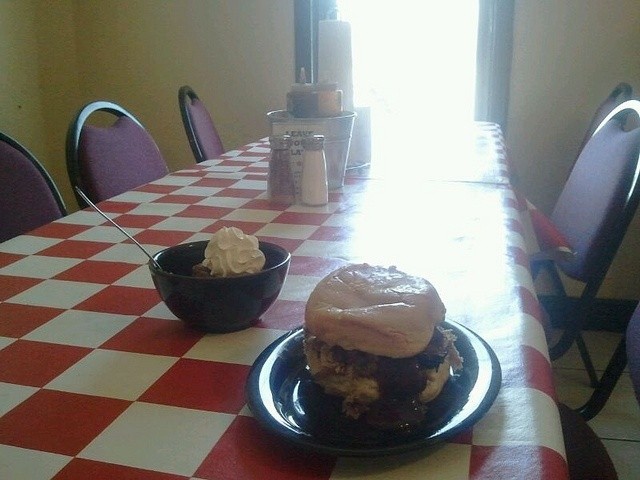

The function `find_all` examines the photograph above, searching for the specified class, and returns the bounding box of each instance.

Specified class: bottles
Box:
[287,67,320,118]
[268,135,296,205]
[301,135,328,207]
[316,69,344,117]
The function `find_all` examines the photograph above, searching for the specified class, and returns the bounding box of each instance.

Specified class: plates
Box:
[245,319,502,458]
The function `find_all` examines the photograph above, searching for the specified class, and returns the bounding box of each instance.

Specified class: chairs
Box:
[531,82,630,388]
[65,100,170,211]
[531,99,640,361]
[0,132,69,243]
[179,85,225,164]
[558,303,640,480]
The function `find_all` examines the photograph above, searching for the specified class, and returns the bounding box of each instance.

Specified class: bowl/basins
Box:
[148,240,291,333]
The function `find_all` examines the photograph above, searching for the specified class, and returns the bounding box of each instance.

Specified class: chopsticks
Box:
[345,106,371,170]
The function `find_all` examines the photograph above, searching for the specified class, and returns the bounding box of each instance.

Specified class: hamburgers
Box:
[303,262,463,430]
